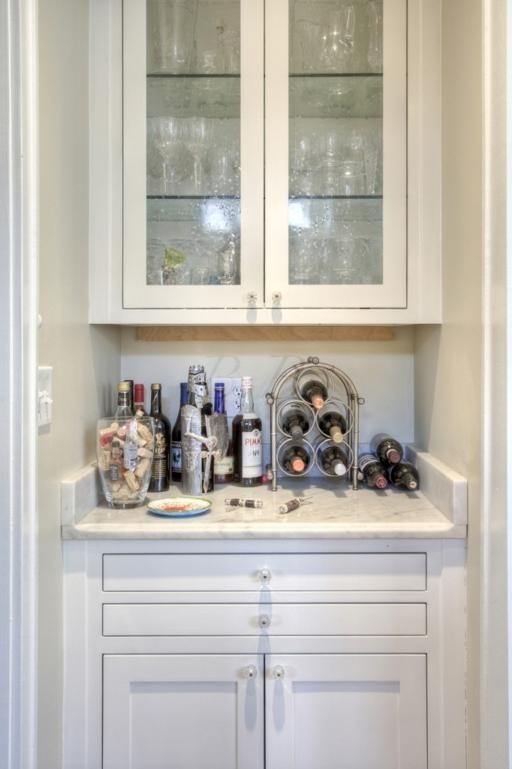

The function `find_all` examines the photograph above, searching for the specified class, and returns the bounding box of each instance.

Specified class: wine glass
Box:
[147,1,380,286]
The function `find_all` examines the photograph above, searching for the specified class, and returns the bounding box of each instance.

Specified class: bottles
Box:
[313,435,351,478]
[114,363,265,495]
[292,368,328,408]
[383,465,419,490]
[358,453,388,490]
[315,396,347,444]
[275,398,309,440]
[369,434,404,467]
[276,435,312,476]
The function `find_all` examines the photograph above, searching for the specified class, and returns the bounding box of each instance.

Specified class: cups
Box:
[95,416,155,510]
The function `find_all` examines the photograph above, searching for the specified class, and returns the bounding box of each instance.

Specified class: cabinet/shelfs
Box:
[62,538,472,769]
[86,0,446,323]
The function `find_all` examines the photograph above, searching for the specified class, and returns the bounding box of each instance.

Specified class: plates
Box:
[147,496,213,516]
